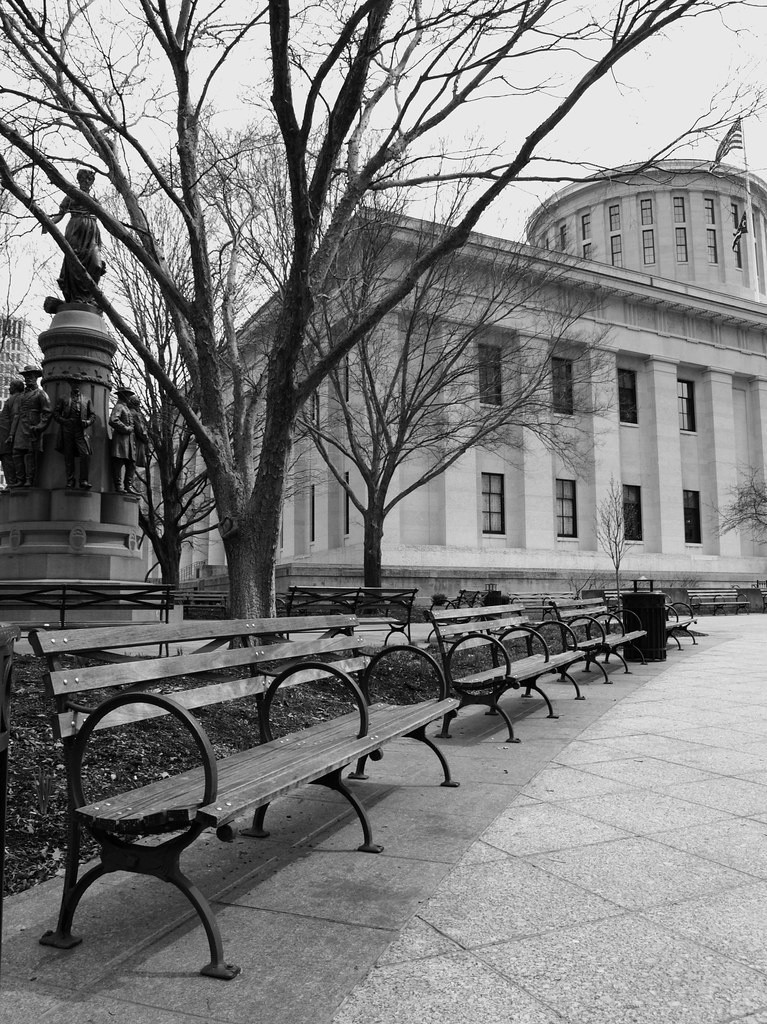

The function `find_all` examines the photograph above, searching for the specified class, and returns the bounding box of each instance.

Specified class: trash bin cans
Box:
[620,576,667,662]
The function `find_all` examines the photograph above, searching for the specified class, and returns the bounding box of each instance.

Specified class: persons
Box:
[0,365,52,489]
[108,385,150,495]
[38,168,107,308]
[53,374,98,490]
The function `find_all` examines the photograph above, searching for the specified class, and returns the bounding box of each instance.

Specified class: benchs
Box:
[0,585,767,979]
